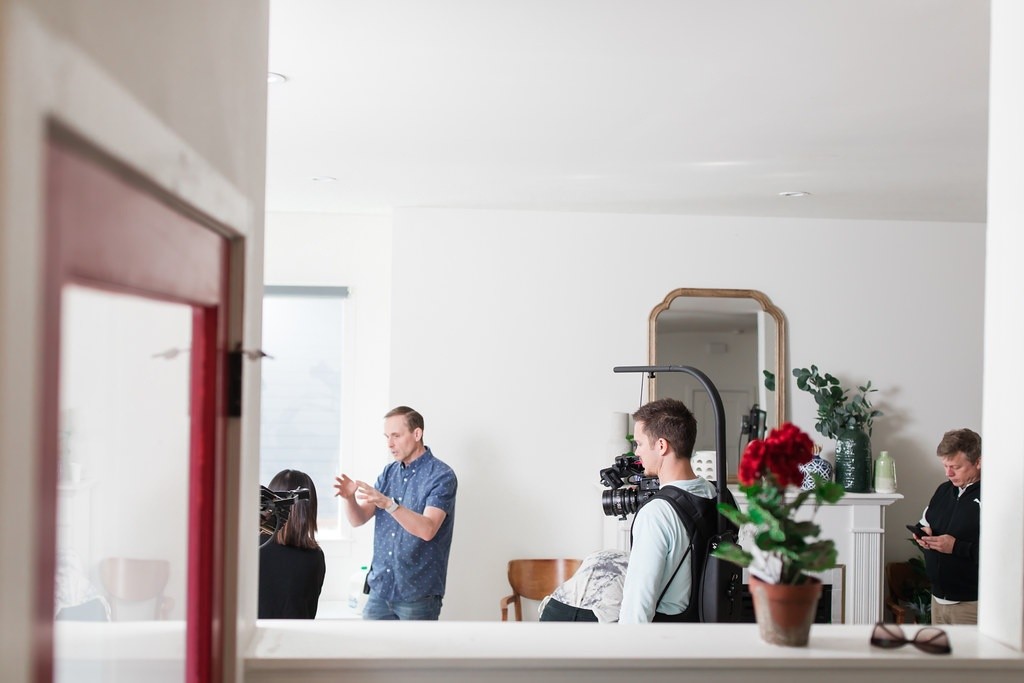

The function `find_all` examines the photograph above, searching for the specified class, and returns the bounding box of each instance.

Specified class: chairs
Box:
[501,558,582,624]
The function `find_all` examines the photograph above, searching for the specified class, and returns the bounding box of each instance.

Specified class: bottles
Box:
[874,450,897,494]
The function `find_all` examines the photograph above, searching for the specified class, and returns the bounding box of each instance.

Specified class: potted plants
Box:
[793,365,884,493]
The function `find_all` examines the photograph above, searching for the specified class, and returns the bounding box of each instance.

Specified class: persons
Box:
[257,469,327,619]
[334,406,458,621]
[913,428,982,625]
[618,399,741,623]
[538,549,632,622]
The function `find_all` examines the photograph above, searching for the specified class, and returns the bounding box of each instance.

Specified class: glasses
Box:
[871,621,951,655]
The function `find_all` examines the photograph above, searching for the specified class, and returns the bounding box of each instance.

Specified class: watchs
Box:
[386,497,399,514]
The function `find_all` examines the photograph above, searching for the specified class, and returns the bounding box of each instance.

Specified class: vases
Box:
[750,575,822,647]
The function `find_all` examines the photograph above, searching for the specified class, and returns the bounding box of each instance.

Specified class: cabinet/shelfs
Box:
[602,484,904,626]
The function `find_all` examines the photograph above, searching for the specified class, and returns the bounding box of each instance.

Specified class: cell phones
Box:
[906,525,930,540]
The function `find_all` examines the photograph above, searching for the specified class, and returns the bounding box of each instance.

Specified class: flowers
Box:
[709,422,844,584]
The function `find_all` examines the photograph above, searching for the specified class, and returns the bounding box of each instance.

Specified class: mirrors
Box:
[647,288,786,484]
